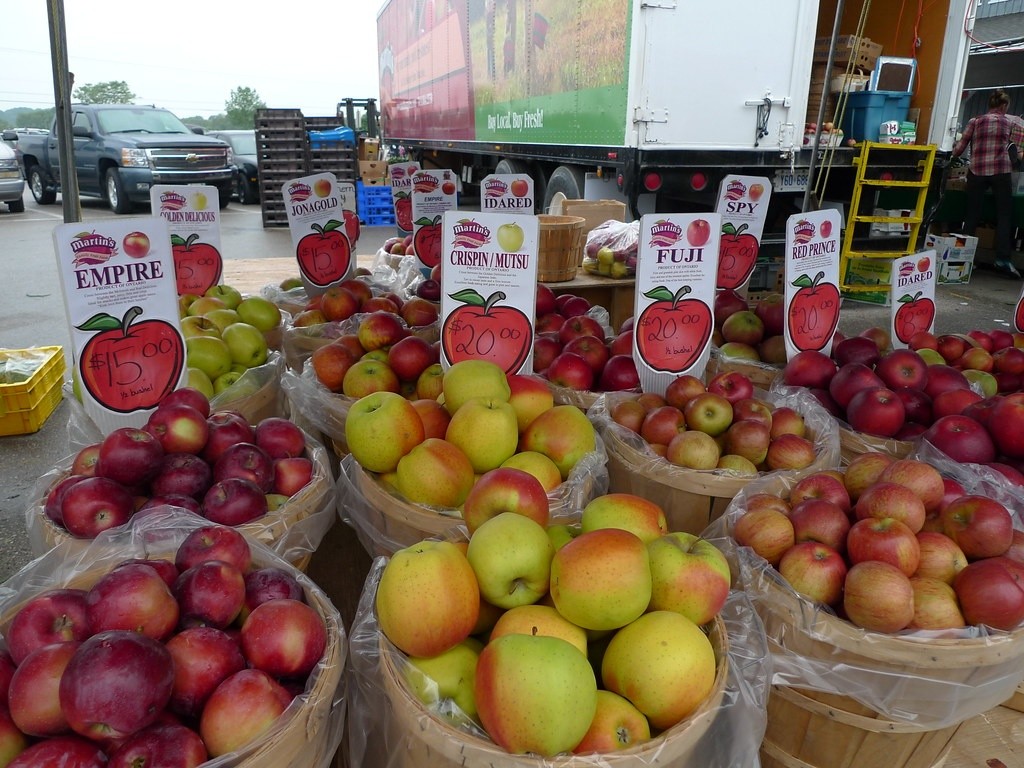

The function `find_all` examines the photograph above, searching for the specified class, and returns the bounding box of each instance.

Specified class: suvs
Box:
[0,127,50,147]
[205,130,260,204]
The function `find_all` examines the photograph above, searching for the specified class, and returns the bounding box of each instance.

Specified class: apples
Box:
[802,122,857,147]
[496,221,524,251]
[749,184,763,200]
[442,181,456,195]
[687,218,710,246]
[279,234,731,757]
[407,165,418,176]
[917,256,930,271]
[123,232,151,258]
[314,178,331,197]
[511,178,528,197]
[609,291,1024,637]
[820,221,832,238]
[0,285,327,768]
[191,191,207,209]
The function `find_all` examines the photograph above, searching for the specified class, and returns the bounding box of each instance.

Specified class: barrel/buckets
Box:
[0,214,1023,768]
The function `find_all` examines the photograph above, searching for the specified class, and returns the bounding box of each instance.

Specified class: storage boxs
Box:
[833,91,914,146]
[871,208,917,232]
[814,49,877,74]
[840,250,899,307]
[561,199,626,267]
[254,107,396,229]
[815,34,884,58]
[924,233,978,262]
[869,230,911,238]
[0,346,66,436]
[830,74,870,93]
[936,261,973,284]
[803,65,855,147]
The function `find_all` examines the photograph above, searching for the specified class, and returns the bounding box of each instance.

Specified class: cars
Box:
[0,129,26,213]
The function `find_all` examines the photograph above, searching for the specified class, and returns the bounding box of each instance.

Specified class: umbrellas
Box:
[1003,113,1024,146]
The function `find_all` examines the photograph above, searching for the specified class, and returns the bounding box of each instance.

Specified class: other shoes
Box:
[993,256,1021,279]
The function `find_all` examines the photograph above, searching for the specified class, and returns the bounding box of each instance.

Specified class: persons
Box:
[1004,141,1018,167]
[952,89,1024,277]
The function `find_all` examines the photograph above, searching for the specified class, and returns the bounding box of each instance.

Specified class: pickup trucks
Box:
[15,103,238,215]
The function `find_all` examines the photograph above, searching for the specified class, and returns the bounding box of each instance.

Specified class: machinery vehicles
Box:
[336,97,381,159]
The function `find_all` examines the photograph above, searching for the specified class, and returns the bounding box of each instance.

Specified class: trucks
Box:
[379,1,979,218]
